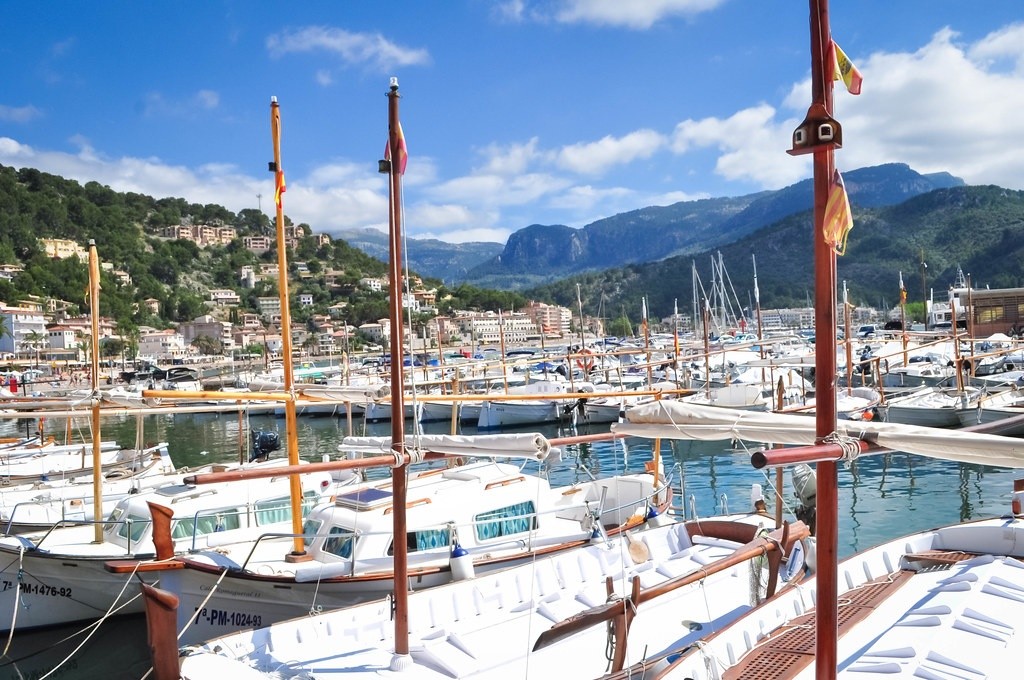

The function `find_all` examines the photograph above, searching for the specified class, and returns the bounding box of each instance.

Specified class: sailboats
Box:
[1,238,1022,640]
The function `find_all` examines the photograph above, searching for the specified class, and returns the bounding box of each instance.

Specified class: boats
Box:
[646,0,1024,680]
[141,72,812,680]
[102,88,679,649]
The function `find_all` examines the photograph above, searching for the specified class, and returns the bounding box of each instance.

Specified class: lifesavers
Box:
[576,348,594,369]
